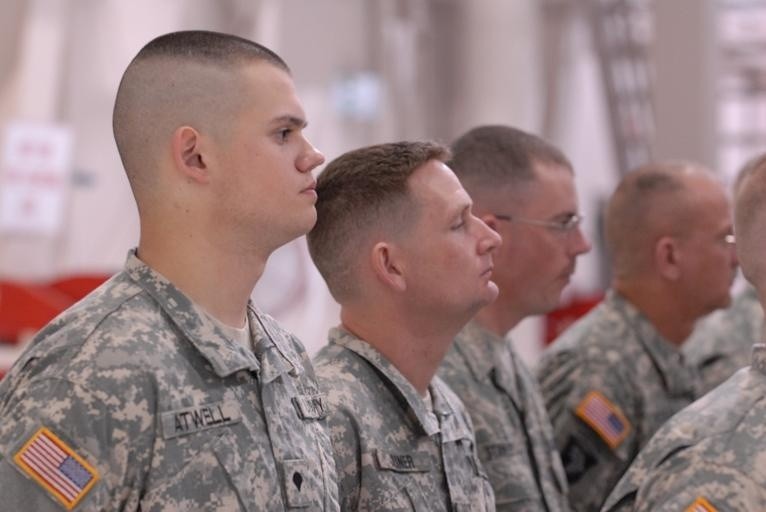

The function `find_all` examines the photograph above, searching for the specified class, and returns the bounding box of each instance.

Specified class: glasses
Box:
[493,213,583,231]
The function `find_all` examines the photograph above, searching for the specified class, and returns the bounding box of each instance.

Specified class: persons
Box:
[684,285,764,388]
[429,121,592,512]
[0,26,340,511]
[305,139,507,511]
[595,149,766,512]
[532,157,739,512]
